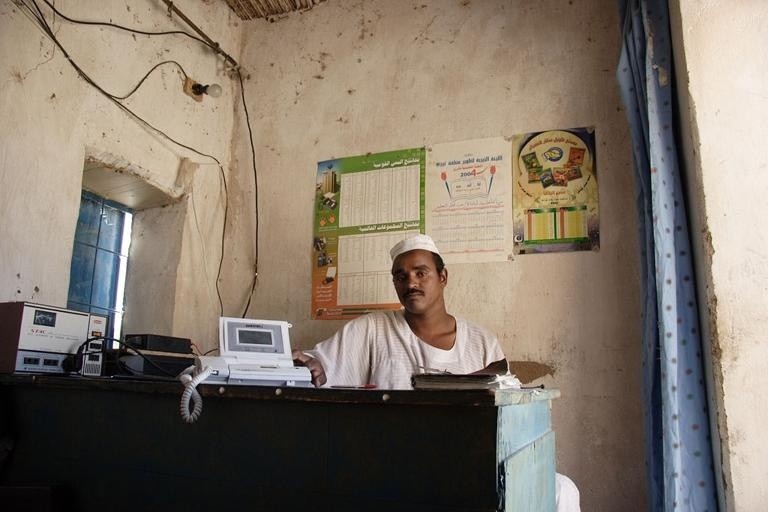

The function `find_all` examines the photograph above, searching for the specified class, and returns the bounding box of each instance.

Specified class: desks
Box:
[2,372,557,511]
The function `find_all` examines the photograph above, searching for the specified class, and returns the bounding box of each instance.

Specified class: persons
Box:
[295,233,580,512]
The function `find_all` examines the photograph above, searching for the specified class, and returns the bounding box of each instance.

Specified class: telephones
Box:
[193,317,312,388]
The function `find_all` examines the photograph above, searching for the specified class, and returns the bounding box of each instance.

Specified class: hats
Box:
[390,235,441,265]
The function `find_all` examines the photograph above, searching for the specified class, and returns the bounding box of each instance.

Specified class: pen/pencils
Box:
[330,384,376,390]
